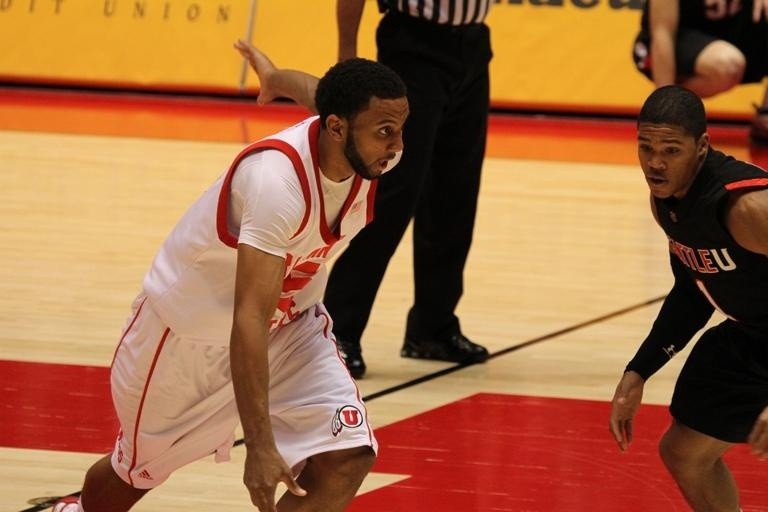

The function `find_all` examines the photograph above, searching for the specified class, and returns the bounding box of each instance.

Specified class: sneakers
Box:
[339,339,367,379]
[399,327,488,363]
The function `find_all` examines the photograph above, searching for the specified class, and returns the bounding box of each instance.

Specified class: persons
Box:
[324,0,494,379]
[608,86,768,511]
[21,39,411,511]
[633,0,768,145]
